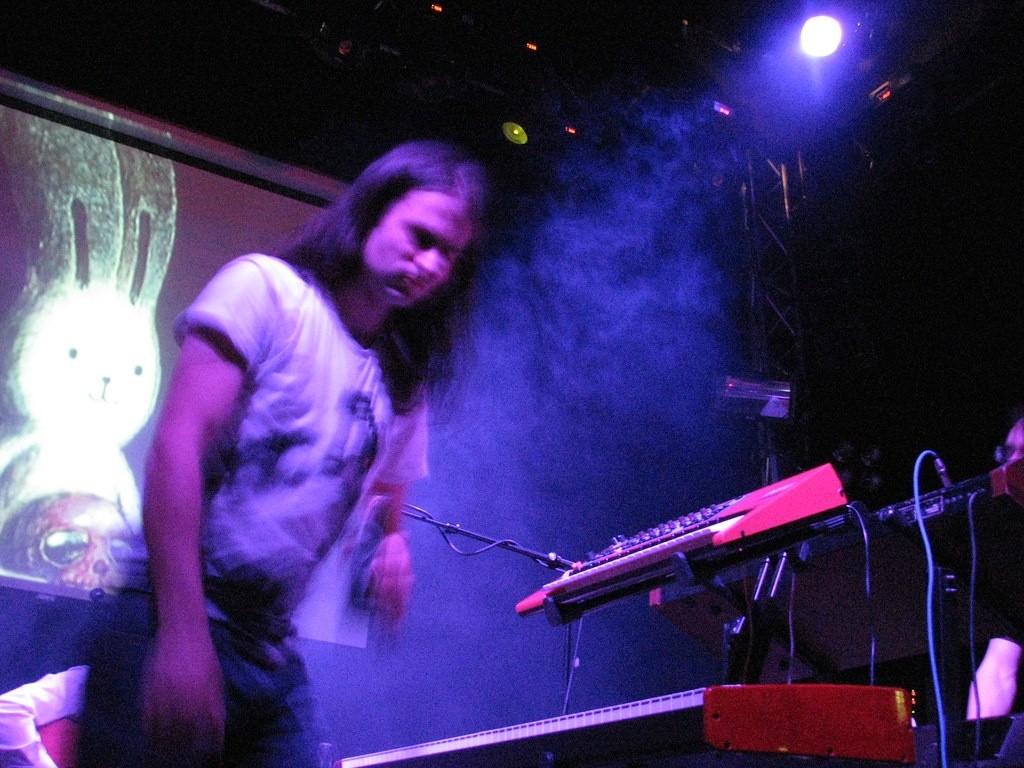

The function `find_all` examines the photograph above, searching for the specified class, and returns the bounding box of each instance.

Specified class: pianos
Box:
[335,683,918,768]
[512,462,854,619]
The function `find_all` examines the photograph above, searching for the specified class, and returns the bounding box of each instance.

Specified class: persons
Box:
[0,665,91,768]
[79,141,490,766]
[967,416,1024,719]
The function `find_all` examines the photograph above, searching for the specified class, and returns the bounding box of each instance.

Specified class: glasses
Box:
[995,445,1024,463]
[10,525,134,572]
